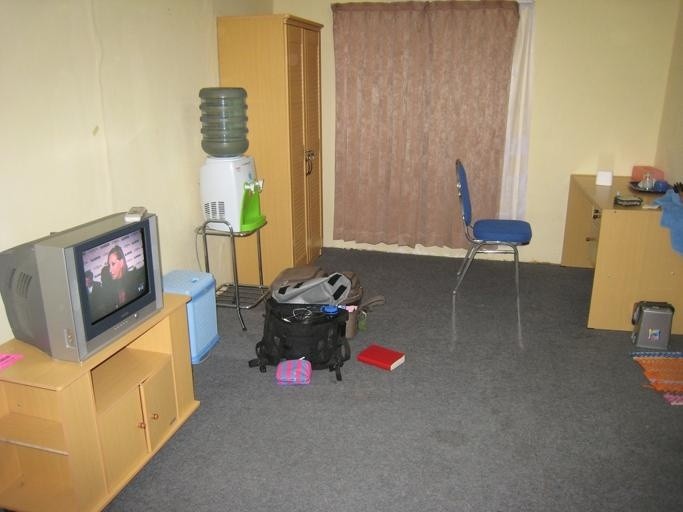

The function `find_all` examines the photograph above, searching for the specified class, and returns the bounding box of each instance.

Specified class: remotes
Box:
[125,206,147,222]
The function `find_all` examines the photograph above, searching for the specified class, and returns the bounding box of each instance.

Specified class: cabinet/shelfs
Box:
[218,13,324,288]
[0,293,201,512]
[195,219,271,330]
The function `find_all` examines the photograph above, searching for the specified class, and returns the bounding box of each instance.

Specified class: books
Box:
[356,343,405,372]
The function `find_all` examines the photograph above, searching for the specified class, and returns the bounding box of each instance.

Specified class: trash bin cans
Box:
[163,269,221,365]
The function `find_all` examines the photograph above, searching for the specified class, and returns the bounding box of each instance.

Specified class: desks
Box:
[561,175,683,335]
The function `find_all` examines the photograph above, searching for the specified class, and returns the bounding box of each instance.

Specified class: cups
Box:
[642,174,654,190]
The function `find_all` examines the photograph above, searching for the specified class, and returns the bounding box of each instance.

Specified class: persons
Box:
[85,245,147,324]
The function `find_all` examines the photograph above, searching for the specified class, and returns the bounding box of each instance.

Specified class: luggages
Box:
[270,267,369,339]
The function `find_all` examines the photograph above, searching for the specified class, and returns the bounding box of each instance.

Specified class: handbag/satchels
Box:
[249,297,350,381]
[276,360,312,385]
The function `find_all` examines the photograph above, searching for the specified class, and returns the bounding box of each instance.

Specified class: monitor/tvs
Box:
[0,212,164,363]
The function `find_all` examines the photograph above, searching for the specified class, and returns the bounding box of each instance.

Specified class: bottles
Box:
[320,304,340,318]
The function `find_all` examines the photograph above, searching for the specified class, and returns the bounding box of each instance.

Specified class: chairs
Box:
[453,159,532,294]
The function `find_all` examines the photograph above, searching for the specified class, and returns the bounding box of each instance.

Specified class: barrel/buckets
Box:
[199,87,248,157]
